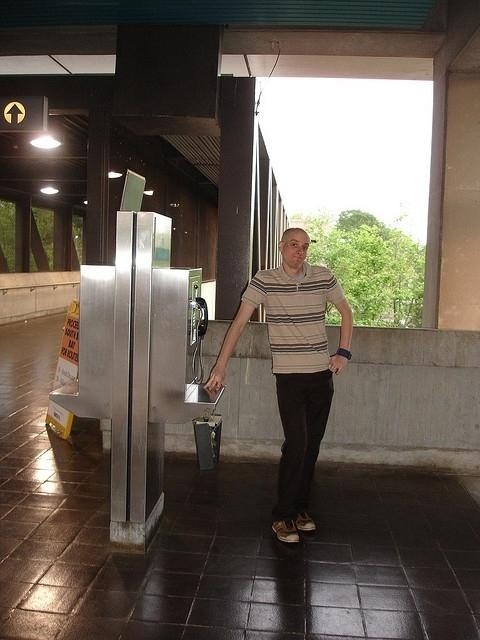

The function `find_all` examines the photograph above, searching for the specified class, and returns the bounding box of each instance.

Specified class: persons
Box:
[200,226,356,546]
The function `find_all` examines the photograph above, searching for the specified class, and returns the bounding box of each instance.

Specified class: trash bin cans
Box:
[191,416,223,473]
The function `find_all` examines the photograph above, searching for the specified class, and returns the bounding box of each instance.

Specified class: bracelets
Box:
[336,347,353,361]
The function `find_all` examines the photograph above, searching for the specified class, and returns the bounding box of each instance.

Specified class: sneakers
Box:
[272,517,299,542]
[294,510,316,531]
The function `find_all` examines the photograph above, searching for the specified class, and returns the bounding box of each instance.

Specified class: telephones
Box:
[196,297,209,336]
